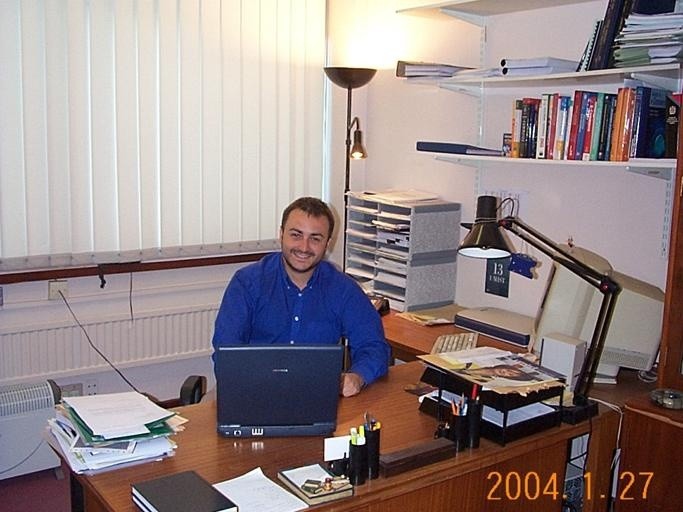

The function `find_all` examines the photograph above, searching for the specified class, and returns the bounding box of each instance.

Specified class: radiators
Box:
[0,304,221,385]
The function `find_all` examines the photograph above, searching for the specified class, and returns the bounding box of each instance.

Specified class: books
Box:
[277,463,353,505]
[503,86,683,160]
[374,190,440,204]
[500,56,579,76]
[576,1,683,72]
[48,392,238,512]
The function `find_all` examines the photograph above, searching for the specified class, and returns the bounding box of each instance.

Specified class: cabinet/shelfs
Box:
[395,0,683,175]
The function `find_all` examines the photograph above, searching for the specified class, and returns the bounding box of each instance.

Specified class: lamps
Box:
[456,194,622,424]
[324,67,378,277]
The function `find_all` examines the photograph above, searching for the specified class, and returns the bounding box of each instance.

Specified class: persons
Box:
[451,364,532,382]
[212,197,392,397]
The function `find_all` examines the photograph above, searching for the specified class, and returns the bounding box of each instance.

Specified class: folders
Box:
[417,141,504,156]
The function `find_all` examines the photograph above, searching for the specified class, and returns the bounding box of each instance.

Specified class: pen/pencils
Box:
[350,410,381,445]
[451,384,482,416]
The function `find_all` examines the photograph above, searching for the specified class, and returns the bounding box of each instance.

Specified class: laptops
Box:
[215,345,346,439]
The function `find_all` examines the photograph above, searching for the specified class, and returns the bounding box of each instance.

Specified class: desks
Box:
[44,302,657,511]
